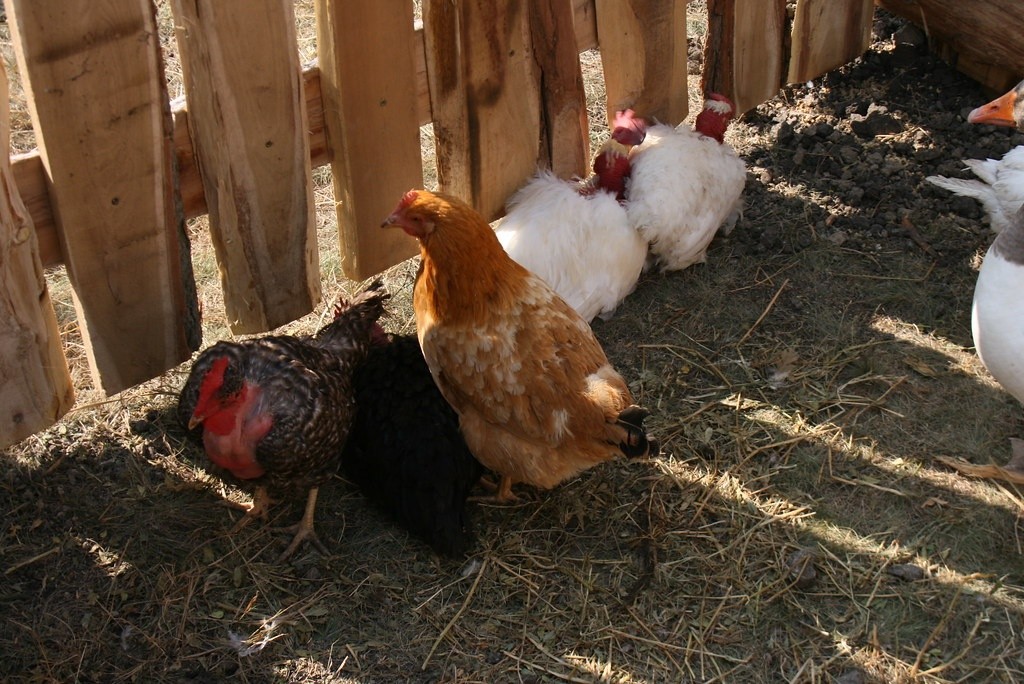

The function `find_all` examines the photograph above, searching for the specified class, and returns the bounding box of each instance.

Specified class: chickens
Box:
[174,91,748,570]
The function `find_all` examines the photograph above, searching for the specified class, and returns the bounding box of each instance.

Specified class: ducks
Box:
[922,77,1024,471]
